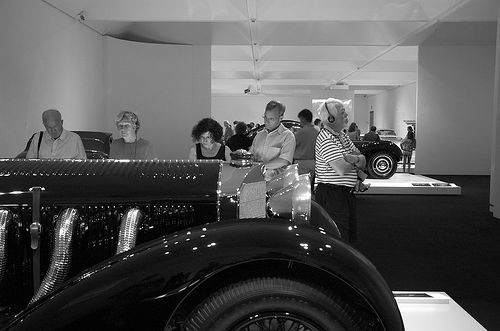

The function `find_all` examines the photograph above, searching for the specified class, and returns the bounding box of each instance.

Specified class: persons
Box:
[24,109,88,159]
[313,97,367,247]
[247,100,297,184]
[312,119,321,133]
[187,117,233,161]
[363,126,381,141]
[401,126,414,163]
[400,131,416,173]
[109,111,159,159]
[346,122,360,141]
[223,119,261,151]
[355,125,361,141]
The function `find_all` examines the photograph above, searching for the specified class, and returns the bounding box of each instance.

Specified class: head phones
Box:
[325,102,336,124]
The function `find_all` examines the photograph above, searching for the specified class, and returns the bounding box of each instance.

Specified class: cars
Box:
[69,130,113,160]
[0,149,405,331]
[225,119,404,180]
[377,128,403,143]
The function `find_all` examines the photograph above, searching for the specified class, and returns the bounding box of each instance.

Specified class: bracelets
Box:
[354,155,360,164]
